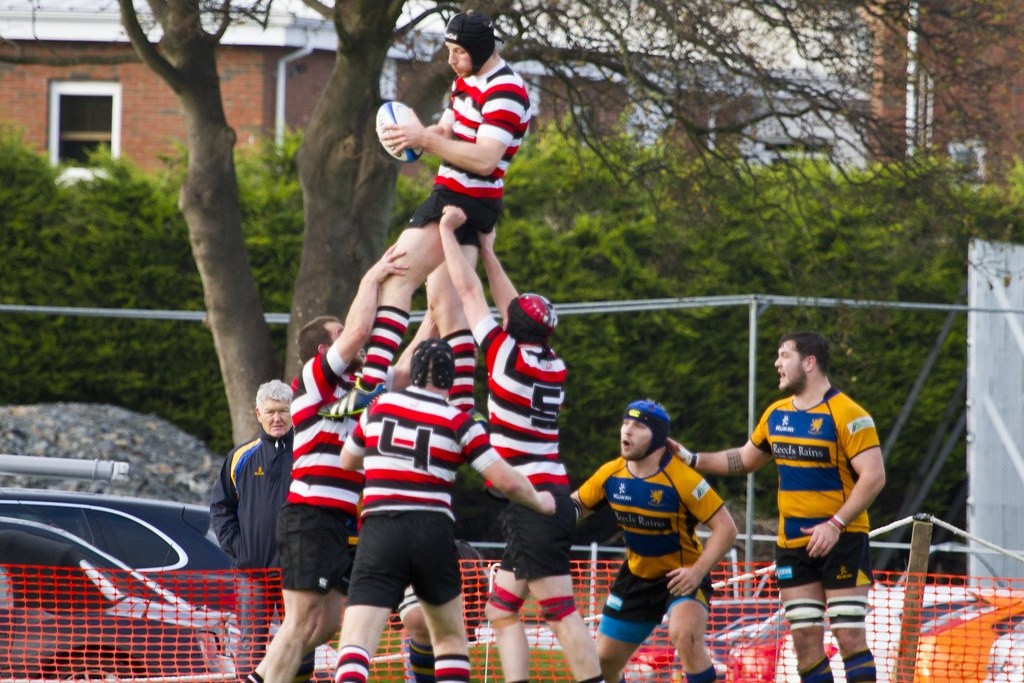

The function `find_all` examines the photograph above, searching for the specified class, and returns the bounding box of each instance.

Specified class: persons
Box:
[210,205,608,683]
[316,9,531,428]
[570,399,738,683]
[666,331,888,683]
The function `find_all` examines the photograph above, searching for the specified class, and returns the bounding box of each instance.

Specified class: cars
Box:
[467,564,1024,683]
[0,483,286,683]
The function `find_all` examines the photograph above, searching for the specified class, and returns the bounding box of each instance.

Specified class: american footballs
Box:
[376,102,423,163]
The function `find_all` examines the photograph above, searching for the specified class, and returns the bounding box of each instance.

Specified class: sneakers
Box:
[317,376,384,418]
[466,407,487,426]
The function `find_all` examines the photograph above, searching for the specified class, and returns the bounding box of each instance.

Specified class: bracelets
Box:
[827,514,847,533]
[684,453,698,467]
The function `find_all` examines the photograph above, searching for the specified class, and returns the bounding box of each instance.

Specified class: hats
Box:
[443,8,496,75]
[504,292,559,344]
[621,398,672,461]
[408,337,457,391]
